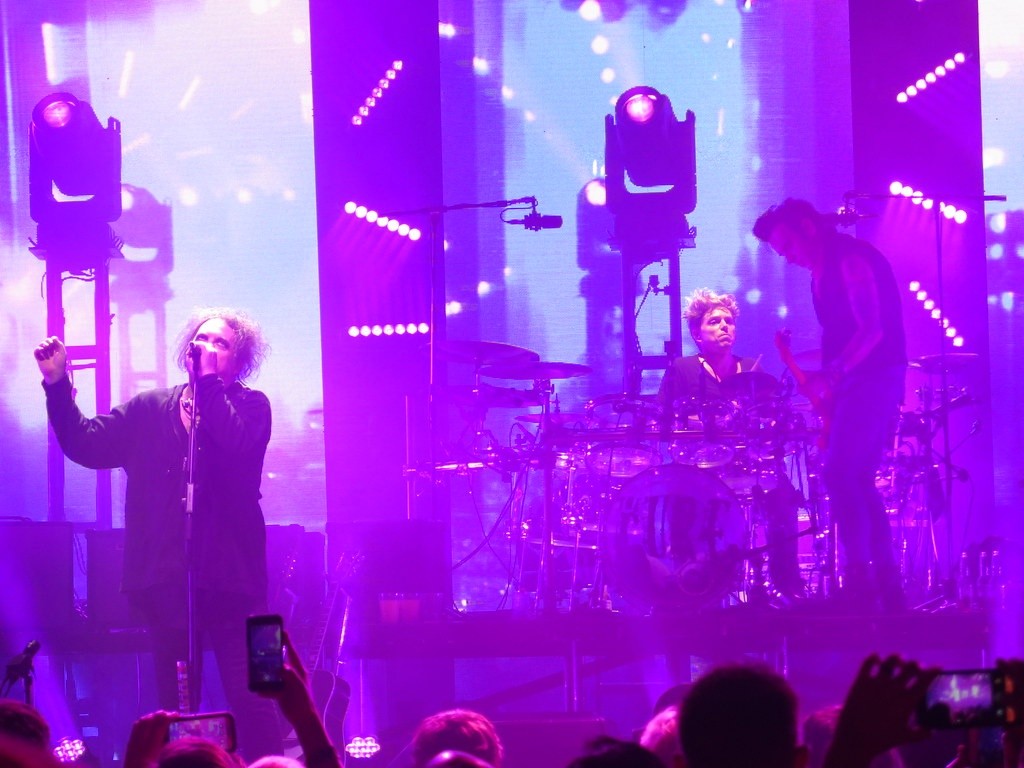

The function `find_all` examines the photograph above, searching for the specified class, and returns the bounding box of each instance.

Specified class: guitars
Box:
[775,326,833,451]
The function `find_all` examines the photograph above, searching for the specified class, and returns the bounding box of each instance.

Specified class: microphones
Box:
[823,209,875,226]
[189,342,201,357]
[510,215,563,229]
[681,566,715,593]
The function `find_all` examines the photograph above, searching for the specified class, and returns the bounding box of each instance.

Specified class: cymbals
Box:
[477,362,589,381]
[513,411,593,424]
[795,348,822,362]
[719,371,778,398]
[445,384,545,407]
[426,337,540,365]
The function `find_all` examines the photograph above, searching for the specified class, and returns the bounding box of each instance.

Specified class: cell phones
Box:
[918,668,1006,726]
[245,615,285,691]
[164,711,237,753]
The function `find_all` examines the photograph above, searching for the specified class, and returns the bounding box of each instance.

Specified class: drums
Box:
[584,394,665,478]
[600,463,748,614]
[671,392,740,467]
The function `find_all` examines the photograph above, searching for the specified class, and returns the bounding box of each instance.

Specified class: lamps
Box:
[614,85,686,186]
[573,177,619,272]
[108,182,161,248]
[33,91,108,197]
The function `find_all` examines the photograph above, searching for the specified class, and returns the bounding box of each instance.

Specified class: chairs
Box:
[0,515,326,716]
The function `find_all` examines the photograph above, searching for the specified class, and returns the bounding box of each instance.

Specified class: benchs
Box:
[345,602,998,748]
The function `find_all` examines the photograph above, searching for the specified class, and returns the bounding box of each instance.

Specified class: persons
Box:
[0,629,1024,768]
[33,308,286,760]
[752,198,905,612]
[659,290,799,600]
